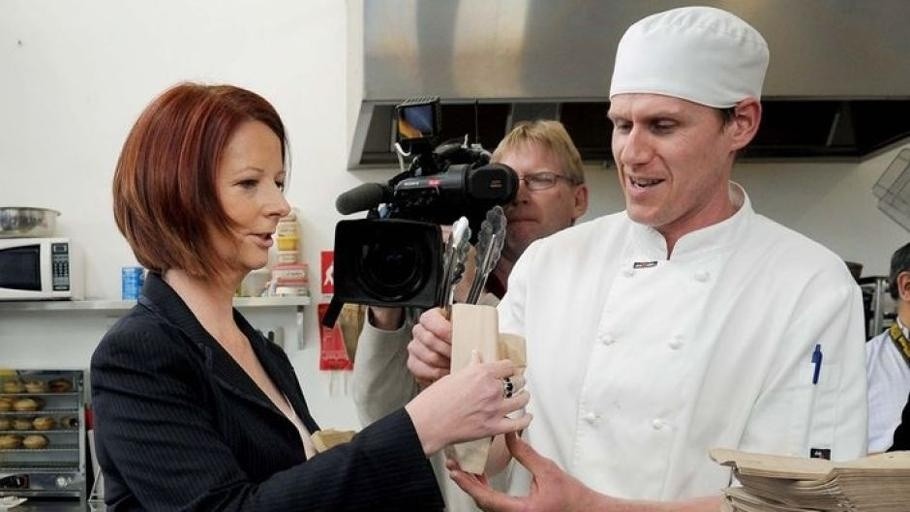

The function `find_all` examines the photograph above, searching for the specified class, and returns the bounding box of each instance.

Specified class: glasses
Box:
[518,172,576,191]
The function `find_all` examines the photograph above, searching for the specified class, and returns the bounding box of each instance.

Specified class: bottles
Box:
[277,215,302,251]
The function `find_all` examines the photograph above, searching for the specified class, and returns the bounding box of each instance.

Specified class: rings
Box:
[502,377,513,399]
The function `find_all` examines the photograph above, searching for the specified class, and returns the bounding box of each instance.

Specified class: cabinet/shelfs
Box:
[0,366,90,511]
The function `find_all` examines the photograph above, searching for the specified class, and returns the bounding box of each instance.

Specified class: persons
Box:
[350,118,590,512]
[406,2,871,512]
[88,80,534,511]
[863,240,909,457]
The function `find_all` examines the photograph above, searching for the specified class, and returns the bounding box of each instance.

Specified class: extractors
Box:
[347,0,909,167]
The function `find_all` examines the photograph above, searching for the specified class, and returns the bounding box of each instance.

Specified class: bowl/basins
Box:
[0,207,64,238]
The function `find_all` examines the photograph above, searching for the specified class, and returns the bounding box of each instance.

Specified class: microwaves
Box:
[0,238,73,301]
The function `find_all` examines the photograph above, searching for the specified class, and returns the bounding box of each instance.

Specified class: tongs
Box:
[439,203,509,320]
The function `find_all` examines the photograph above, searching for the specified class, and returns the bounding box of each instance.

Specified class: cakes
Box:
[0,378,71,450]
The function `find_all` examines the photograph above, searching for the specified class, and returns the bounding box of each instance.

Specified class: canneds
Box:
[121,266,145,301]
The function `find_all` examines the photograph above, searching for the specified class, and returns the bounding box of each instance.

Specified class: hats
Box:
[888,241,910,286]
[608,5,770,111]
